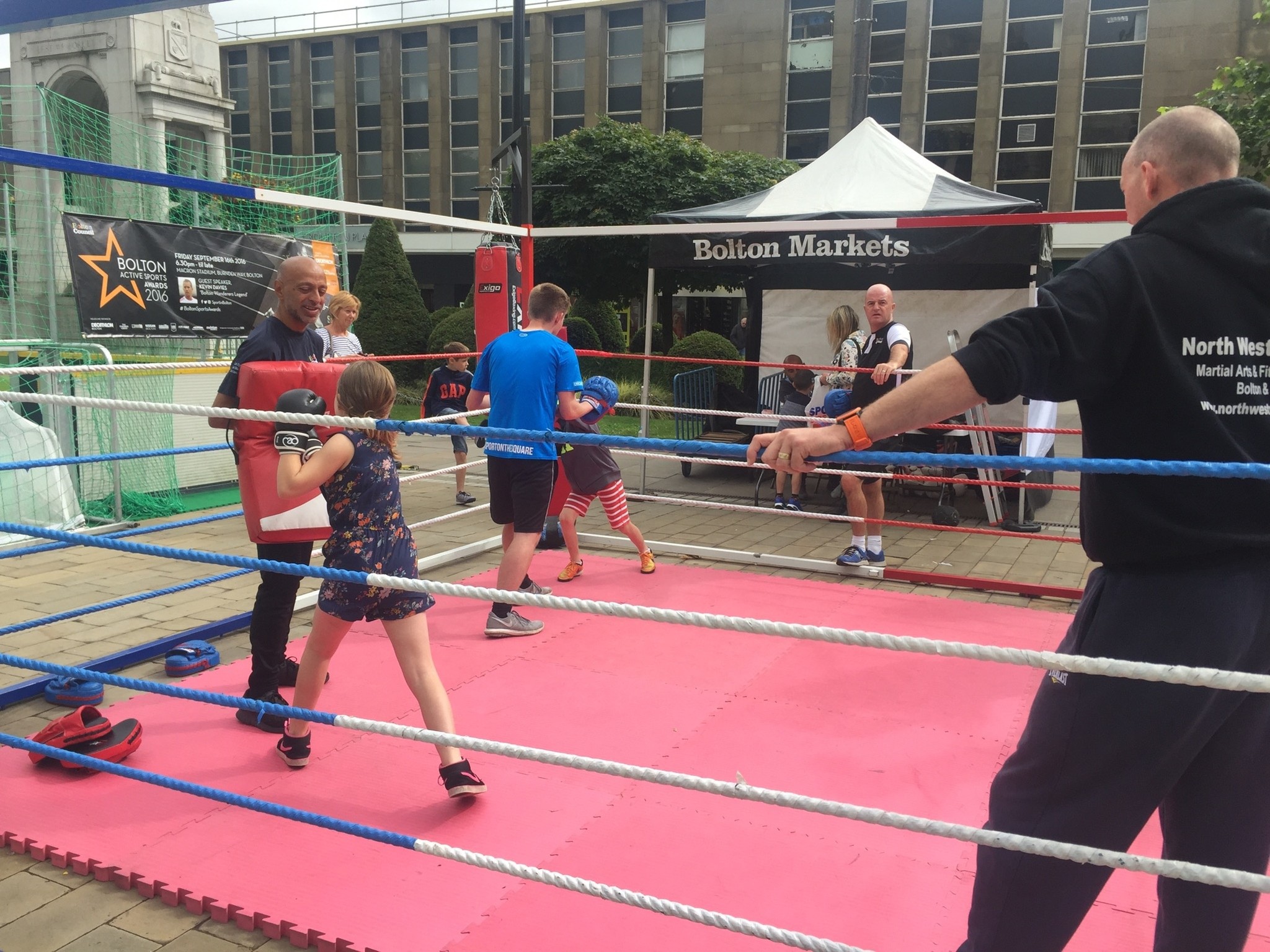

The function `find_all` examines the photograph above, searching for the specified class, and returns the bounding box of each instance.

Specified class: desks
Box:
[736,417,828,508]
[887,428,969,507]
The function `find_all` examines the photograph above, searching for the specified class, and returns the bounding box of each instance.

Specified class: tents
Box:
[646,116,1058,531]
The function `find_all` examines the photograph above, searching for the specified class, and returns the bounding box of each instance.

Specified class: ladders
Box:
[948,328,1004,524]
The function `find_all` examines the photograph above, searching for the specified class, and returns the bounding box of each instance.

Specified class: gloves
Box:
[274,388,326,455]
[301,427,324,466]
[577,376,619,425]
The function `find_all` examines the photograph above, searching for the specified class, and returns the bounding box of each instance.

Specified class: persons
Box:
[820,305,868,522]
[180,279,198,304]
[465,283,619,637]
[671,313,684,339]
[554,402,657,582]
[313,291,373,365]
[779,355,818,406]
[421,341,489,505]
[730,317,746,357]
[747,104,1270,952]
[771,369,814,511]
[275,360,488,799]
[209,256,329,734]
[836,284,913,567]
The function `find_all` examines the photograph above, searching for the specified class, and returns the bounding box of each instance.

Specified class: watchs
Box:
[836,407,873,452]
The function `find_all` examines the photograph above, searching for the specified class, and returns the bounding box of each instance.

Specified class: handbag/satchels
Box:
[319,327,334,358]
[804,375,834,429]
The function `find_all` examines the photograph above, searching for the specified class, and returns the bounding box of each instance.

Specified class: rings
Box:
[777,452,791,459]
[882,370,886,373]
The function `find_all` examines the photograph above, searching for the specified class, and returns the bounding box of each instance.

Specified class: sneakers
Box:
[456,490,476,505]
[514,580,553,606]
[836,545,869,566]
[276,718,311,766]
[437,756,487,799]
[774,497,788,510]
[638,547,656,574]
[484,610,545,637]
[557,557,583,582]
[787,498,804,512]
[865,550,887,567]
[236,688,291,734]
[474,419,488,448]
[279,656,329,687]
